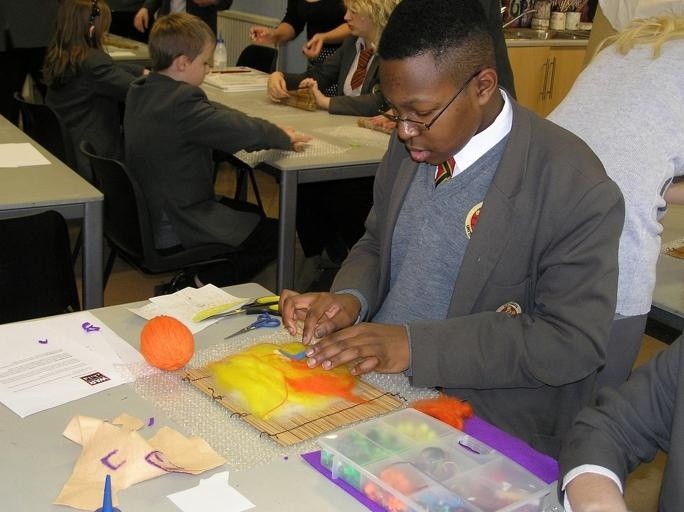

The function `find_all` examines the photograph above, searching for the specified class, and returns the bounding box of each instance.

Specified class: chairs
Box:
[213,44,278,218]
[13,91,66,164]
[73,141,244,296]
[0,210,80,324]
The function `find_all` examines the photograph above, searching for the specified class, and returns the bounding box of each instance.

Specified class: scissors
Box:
[225,314,280,340]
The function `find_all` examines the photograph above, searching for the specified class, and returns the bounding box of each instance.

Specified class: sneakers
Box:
[168,269,197,294]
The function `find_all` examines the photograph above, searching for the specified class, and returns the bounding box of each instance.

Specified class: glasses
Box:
[376,69,481,131]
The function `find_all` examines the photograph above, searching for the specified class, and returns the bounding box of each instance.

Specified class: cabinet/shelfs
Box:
[507,47,586,119]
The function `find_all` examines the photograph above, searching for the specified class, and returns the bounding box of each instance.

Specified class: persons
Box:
[557,333,684,512]
[41,1,404,247]
[544,15,684,394]
[123,12,314,298]
[279,0,625,461]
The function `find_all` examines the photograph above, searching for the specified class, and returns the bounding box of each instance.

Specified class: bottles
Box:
[212,32,228,71]
[94,476,123,512]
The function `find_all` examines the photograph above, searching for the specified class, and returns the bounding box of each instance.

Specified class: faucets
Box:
[501,7,538,28]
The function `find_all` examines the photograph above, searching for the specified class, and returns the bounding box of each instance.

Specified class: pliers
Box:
[221,296,281,316]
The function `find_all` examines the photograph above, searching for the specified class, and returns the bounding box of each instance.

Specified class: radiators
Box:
[217,10,288,72]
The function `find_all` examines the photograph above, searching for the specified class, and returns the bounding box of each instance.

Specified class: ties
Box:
[434,157,455,190]
[351,47,374,90]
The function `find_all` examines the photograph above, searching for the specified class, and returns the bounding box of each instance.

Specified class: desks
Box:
[645,205,683,345]
[24,31,150,104]
[197,65,390,298]
[0,114,107,310]
[0,282,565,512]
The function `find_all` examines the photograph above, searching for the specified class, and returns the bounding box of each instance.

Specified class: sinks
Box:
[504,30,583,39]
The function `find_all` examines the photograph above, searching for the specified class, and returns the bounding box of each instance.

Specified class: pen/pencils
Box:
[210,70,251,73]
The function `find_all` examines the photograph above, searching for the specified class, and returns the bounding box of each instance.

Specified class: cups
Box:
[549,12,566,31]
[567,12,581,30]
